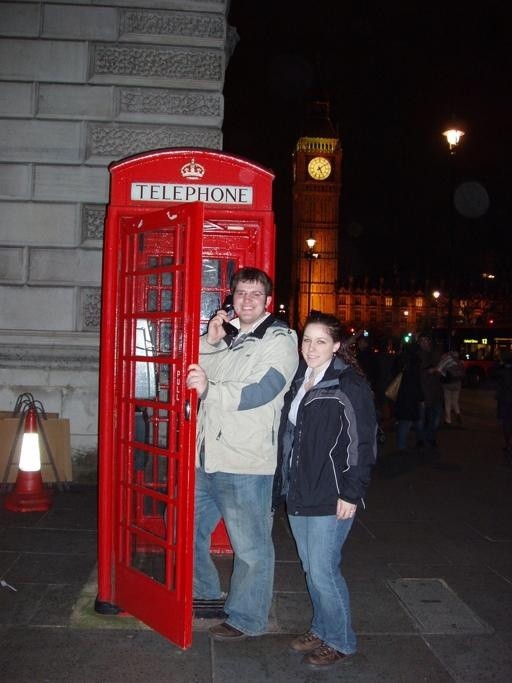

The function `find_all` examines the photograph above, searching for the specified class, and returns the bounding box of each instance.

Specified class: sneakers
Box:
[394,440,443,454]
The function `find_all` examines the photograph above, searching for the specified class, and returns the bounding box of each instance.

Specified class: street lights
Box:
[442,121,466,352]
[305,230,319,321]
[432,290,442,328]
[404,309,409,329]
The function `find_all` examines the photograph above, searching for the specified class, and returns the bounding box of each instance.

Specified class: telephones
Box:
[221,295,239,337]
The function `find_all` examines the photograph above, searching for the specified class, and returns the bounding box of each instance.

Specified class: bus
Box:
[416,325,512,384]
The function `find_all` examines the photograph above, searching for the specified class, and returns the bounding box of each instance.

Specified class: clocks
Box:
[308,155,333,180]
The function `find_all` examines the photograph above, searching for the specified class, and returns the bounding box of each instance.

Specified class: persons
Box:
[185,264,302,641]
[275,309,380,670]
[356,332,512,454]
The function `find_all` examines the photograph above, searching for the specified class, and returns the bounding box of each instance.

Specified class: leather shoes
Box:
[209,623,253,640]
[290,632,354,667]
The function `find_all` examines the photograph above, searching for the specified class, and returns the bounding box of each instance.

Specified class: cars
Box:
[355,327,399,366]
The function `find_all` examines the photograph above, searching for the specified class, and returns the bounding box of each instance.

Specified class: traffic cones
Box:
[4,404,54,514]
